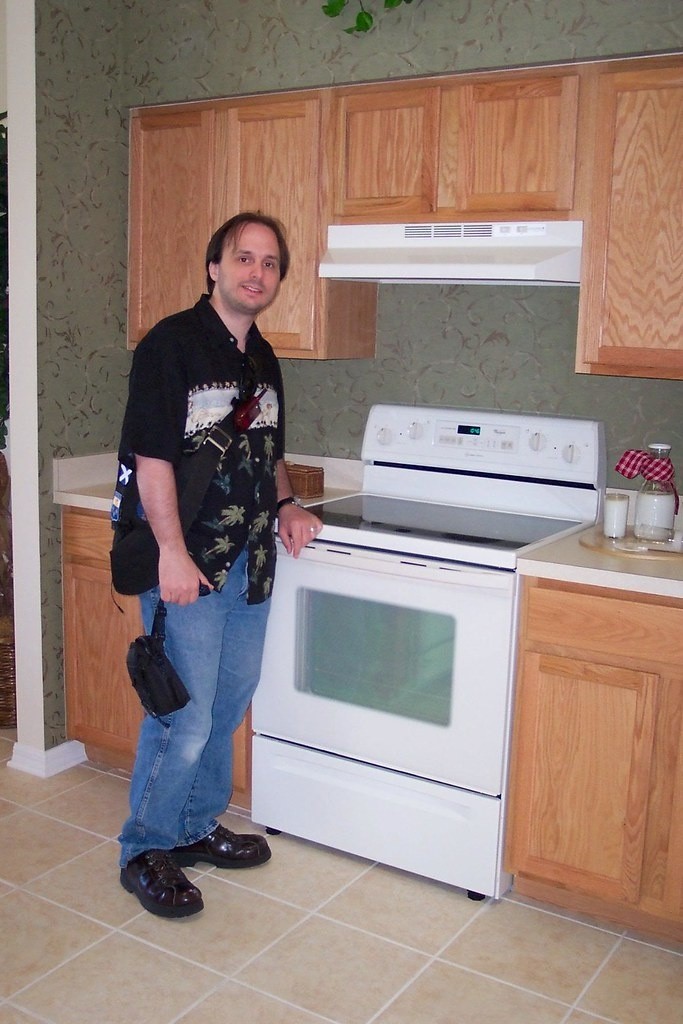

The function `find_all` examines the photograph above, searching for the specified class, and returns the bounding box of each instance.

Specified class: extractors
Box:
[317,220,583,288]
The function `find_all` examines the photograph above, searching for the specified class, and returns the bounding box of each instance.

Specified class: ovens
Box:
[250,536,520,905]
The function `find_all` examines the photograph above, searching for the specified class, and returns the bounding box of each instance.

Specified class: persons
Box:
[107,213,322,918]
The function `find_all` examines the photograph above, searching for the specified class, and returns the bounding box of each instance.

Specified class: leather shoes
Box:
[119,844,204,920]
[169,823,272,868]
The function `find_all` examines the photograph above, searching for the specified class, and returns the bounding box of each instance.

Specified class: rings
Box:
[307,527,317,533]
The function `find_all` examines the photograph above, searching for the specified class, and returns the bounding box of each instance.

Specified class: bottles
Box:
[632,442,676,544]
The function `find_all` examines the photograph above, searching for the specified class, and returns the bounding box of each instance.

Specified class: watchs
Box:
[274,495,303,513]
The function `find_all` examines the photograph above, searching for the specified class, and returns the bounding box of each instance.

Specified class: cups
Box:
[604,493,629,539]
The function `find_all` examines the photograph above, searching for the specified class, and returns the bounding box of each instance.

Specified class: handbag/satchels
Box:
[109,425,237,595]
[126,583,211,729]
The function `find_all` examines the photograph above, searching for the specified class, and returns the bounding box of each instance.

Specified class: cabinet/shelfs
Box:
[127,48,683,379]
[59,506,253,814]
[503,576,683,941]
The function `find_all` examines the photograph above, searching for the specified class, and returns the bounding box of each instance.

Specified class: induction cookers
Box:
[276,404,607,572]
